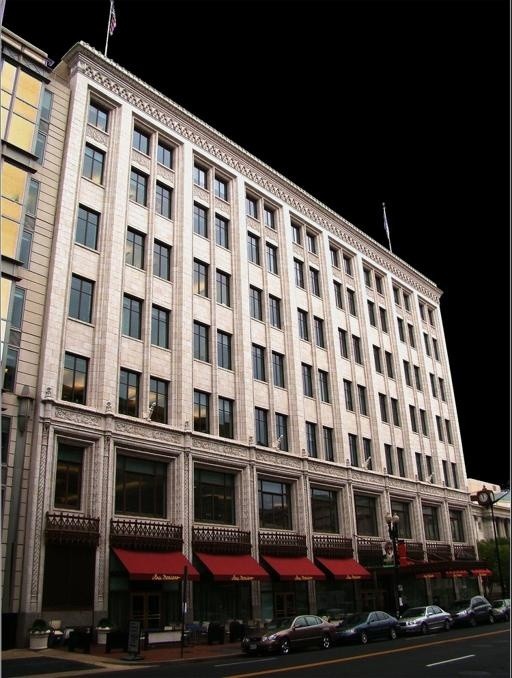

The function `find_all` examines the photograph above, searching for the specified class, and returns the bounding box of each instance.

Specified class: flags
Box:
[383,209,390,238]
[108,4,117,36]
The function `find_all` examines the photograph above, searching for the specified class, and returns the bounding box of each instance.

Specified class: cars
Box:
[240,594,510,657]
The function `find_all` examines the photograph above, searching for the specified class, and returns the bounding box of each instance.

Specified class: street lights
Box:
[384,511,401,618]
[475,485,505,601]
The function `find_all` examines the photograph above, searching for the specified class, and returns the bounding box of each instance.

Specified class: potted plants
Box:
[28,620,53,650]
[95,617,113,644]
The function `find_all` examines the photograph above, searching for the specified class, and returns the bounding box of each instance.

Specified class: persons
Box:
[381,541,395,565]
